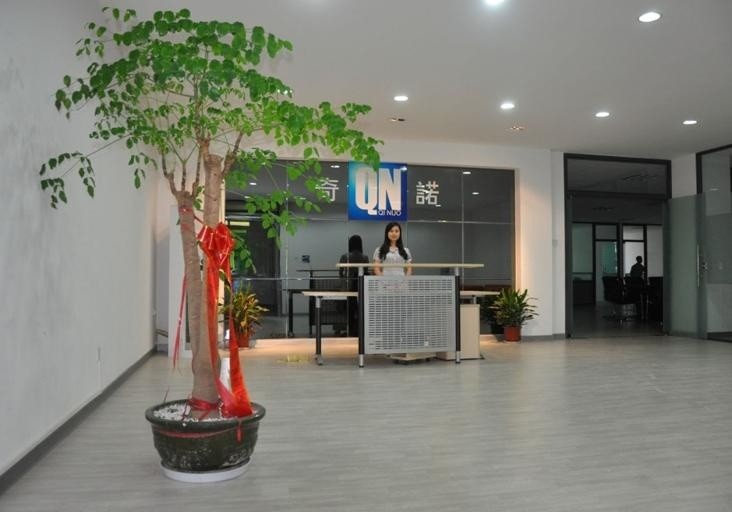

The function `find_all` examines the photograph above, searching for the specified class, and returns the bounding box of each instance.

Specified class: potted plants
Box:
[39,6,385,483]
[494,287,540,341]
[217,280,269,348]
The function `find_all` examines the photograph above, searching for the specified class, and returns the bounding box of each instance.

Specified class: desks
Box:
[302,263,500,368]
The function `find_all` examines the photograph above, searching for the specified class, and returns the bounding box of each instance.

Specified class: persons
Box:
[629,255,644,278]
[374,222,412,293]
[339,235,369,279]
